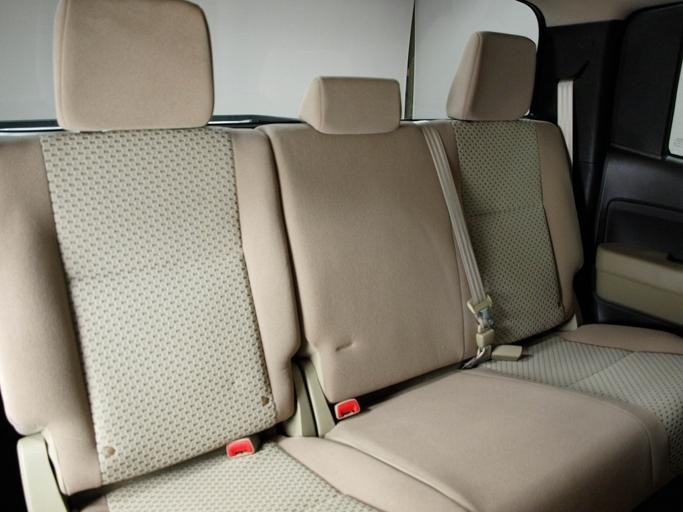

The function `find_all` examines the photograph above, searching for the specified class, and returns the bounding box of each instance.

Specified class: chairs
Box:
[254,75,654,512]
[431,30,683,488]
[0,0,473,512]
